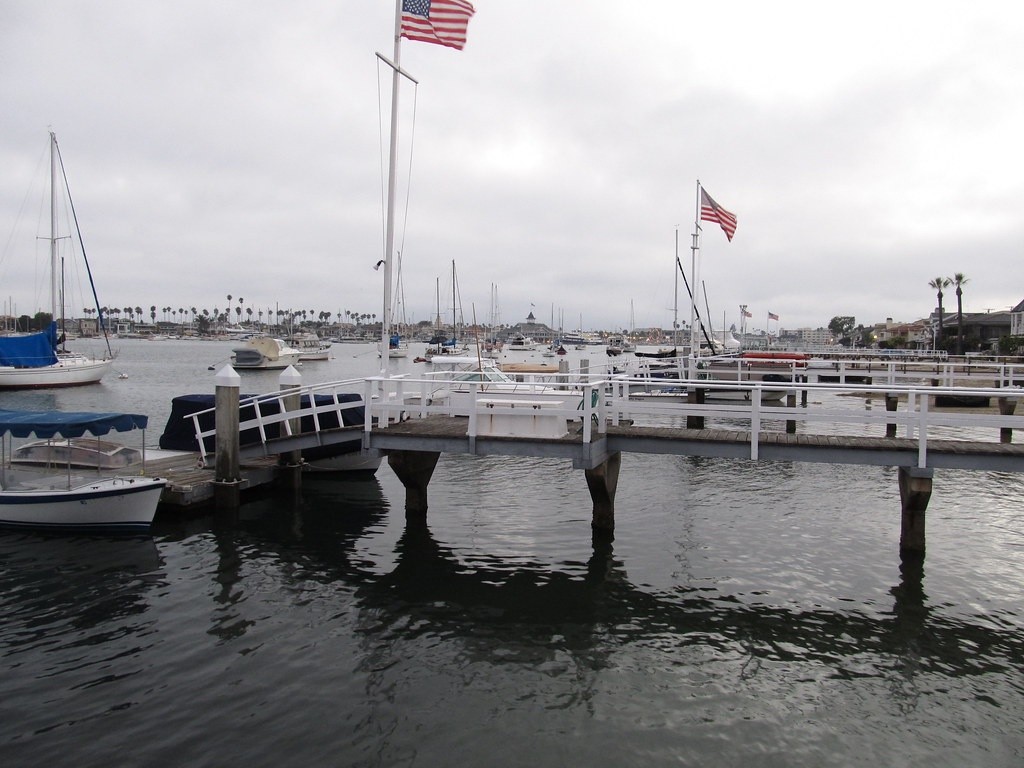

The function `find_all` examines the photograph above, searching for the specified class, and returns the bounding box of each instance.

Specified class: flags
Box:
[740,309,752,317]
[697,188,737,242]
[769,312,778,321]
[402,0,477,51]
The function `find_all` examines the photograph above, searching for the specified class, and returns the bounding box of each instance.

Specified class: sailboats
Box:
[377,249,414,358]
[424,259,470,362]
[623,298,636,352]
[481,283,587,357]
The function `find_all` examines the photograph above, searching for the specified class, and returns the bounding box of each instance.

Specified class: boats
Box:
[164,393,388,481]
[605,336,624,357]
[1,406,167,527]
[633,345,810,400]
[208,336,305,376]
[1,130,121,387]
[282,332,336,361]
